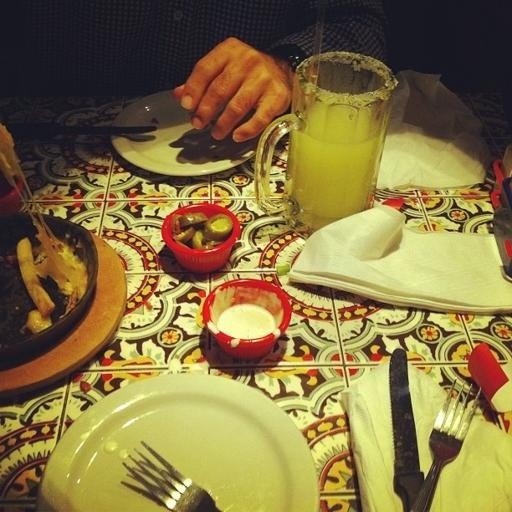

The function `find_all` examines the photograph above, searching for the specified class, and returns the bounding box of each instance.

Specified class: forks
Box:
[420,375,482,504]
[121,440,227,511]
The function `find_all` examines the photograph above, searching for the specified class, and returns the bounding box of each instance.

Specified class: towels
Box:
[287,204,512,314]
[339,357,512,512]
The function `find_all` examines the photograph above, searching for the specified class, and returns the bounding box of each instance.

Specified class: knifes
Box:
[35,119,161,142]
[382,347,427,509]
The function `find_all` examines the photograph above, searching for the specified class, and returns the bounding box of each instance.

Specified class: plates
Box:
[1,211,99,365]
[34,376,325,512]
[109,88,275,176]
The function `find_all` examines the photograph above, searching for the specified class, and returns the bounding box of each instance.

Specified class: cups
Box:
[252,53,397,228]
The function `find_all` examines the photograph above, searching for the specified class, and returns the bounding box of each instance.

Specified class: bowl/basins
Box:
[164,205,241,271]
[204,281,293,353]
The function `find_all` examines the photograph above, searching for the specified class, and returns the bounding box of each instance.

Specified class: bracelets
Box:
[270,41,307,72]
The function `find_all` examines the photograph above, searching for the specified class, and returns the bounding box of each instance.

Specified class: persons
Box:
[0,0,390,144]
